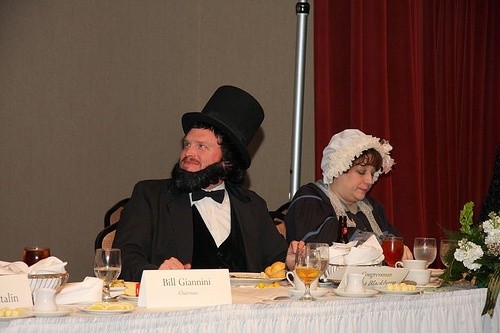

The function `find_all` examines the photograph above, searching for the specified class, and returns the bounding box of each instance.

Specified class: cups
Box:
[32,286,58,312]
[287,268,320,291]
[110,279,125,288]
[123,281,140,296]
[395,258,427,270]
[23,246,50,267]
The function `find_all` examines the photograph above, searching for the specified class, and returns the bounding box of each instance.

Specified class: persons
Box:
[280,129,414,266]
[108,83,311,281]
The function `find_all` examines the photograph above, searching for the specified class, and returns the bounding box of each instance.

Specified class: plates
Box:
[120,294,140,301]
[414,282,440,291]
[230,272,287,282]
[286,285,330,296]
[109,287,126,291]
[32,307,73,316]
[334,287,378,298]
[376,286,424,294]
[78,302,134,315]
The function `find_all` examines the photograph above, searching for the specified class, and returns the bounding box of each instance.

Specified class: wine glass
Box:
[296,248,321,301]
[306,242,329,286]
[440,239,459,269]
[94,248,122,301]
[381,236,405,267]
[414,237,437,269]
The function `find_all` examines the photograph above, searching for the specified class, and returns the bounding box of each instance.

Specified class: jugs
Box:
[344,273,367,294]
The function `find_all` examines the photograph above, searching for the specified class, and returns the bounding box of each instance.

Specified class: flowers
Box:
[440,201,500,319]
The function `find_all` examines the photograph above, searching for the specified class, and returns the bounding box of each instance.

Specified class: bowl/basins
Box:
[403,269,432,285]
[320,251,385,286]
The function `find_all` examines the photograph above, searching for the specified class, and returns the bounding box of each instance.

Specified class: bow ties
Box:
[192,186,225,204]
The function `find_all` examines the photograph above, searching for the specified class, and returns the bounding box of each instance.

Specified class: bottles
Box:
[337,215,350,243]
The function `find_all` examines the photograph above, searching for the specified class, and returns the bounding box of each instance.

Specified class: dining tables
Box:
[2,263,498,333]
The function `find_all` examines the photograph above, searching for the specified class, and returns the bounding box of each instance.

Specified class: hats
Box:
[321,128,396,185]
[181,85,265,169]
